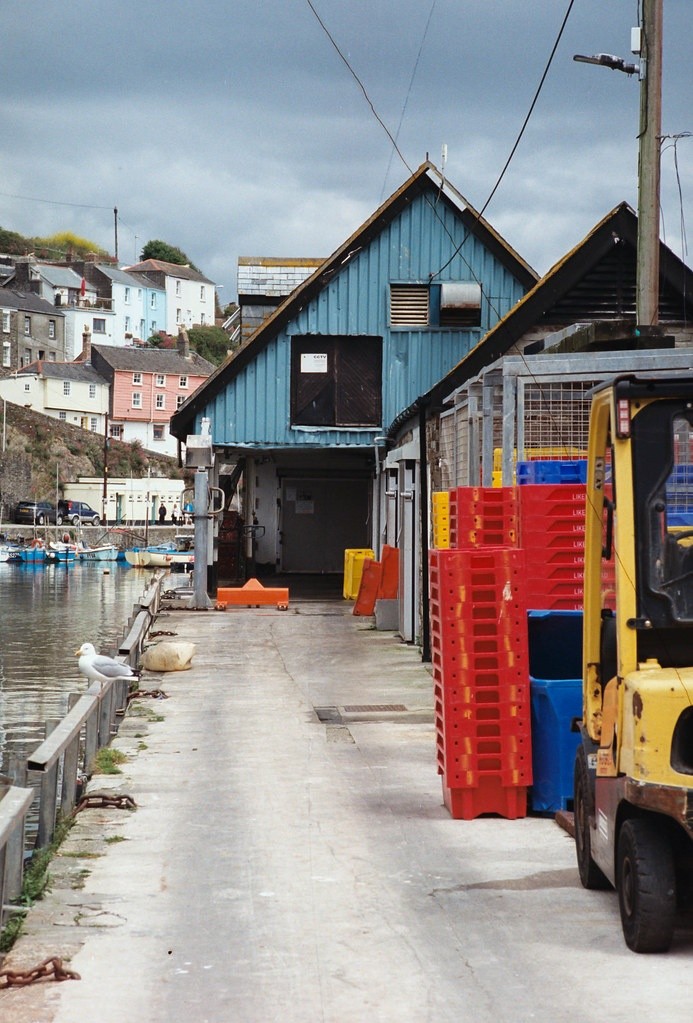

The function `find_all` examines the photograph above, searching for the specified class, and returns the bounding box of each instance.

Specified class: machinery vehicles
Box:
[554,367,693,954]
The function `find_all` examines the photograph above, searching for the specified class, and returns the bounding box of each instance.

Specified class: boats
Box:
[124,470,195,569]
[74,502,118,561]
[0,495,46,562]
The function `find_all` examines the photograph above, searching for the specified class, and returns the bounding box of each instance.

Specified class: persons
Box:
[158,503,166,525]
[184,499,195,512]
[171,504,179,526]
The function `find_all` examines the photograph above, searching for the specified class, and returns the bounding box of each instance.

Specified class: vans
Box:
[15,501,61,525]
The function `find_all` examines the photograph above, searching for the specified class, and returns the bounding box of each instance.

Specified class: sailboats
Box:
[45,463,78,562]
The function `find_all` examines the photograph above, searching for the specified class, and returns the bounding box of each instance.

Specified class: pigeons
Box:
[74,642,141,691]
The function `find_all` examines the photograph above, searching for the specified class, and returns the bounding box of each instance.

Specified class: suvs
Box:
[59,499,100,526]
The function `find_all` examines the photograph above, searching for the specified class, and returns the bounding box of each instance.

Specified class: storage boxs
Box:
[342,441,692,819]
